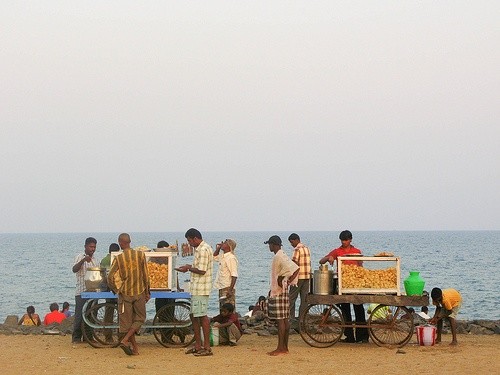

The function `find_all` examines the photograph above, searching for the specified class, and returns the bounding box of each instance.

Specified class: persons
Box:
[246,292,431,322]
[19,305,41,326]
[44,303,67,326]
[100,242,121,343]
[179,228,214,356]
[264,234,300,356]
[72,237,101,345]
[319,230,371,343]
[428,286,462,347]
[288,233,311,323]
[213,238,239,315]
[207,303,245,346]
[61,301,71,319]
[107,233,151,355]
[155,240,185,347]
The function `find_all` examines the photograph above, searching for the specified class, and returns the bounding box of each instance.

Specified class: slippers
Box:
[194,348,214,356]
[120,343,134,355]
[185,347,201,353]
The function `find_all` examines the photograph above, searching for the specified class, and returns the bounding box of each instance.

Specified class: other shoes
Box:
[338,337,355,343]
[356,337,368,343]
[228,342,237,346]
[220,341,228,345]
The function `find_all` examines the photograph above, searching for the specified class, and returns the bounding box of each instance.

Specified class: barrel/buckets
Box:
[416,325,436,347]
[200,327,219,347]
[313,270,334,295]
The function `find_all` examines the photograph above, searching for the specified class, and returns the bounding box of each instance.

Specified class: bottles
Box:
[404,271,425,296]
[85,267,108,292]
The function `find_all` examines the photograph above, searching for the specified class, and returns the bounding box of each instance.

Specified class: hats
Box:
[264,235,283,246]
[225,238,237,255]
